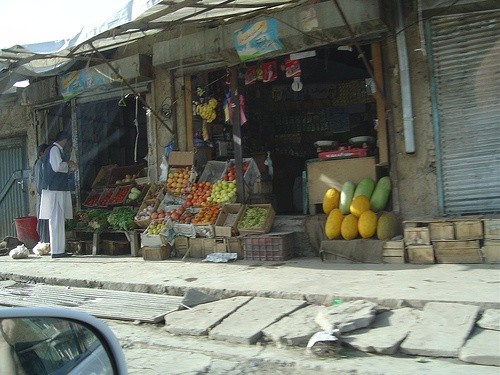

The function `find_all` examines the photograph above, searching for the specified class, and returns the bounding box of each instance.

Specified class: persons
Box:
[32,144,51,242]
[39,131,78,258]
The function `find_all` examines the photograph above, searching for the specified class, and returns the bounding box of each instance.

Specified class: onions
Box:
[134,206,152,220]
[75,211,86,222]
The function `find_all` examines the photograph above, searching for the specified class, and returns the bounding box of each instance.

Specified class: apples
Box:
[193,201,219,224]
[167,167,212,207]
[207,164,248,202]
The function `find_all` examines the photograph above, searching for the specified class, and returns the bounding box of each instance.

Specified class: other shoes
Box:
[52,253,73,258]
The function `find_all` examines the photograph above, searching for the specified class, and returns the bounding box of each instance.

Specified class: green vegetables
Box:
[82,208,138,231]
[66,219,74,225]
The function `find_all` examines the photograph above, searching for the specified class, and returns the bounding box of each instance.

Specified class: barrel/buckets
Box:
[13,216,40,248]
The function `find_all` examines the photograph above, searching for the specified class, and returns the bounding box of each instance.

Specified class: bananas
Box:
[196,98,217,122]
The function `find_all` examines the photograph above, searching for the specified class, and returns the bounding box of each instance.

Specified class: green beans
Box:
[238,207,267,229]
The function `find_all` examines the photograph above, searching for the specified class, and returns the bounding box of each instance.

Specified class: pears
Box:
[145,222,163,235]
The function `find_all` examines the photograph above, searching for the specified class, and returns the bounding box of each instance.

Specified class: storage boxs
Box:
[64,150,297,263]
[382,213,500,264]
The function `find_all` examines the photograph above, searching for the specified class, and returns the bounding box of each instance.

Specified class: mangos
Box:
[151,207,195,224]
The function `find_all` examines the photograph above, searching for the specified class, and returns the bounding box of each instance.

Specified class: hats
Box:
[38,144,49,153]
[54,131,70,142]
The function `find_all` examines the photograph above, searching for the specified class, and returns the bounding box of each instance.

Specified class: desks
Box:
[306,154,388,215]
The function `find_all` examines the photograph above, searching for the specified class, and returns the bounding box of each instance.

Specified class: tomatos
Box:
[86,188,129,206]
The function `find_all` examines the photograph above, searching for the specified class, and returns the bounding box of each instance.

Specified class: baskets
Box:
[244,235,295,260]
